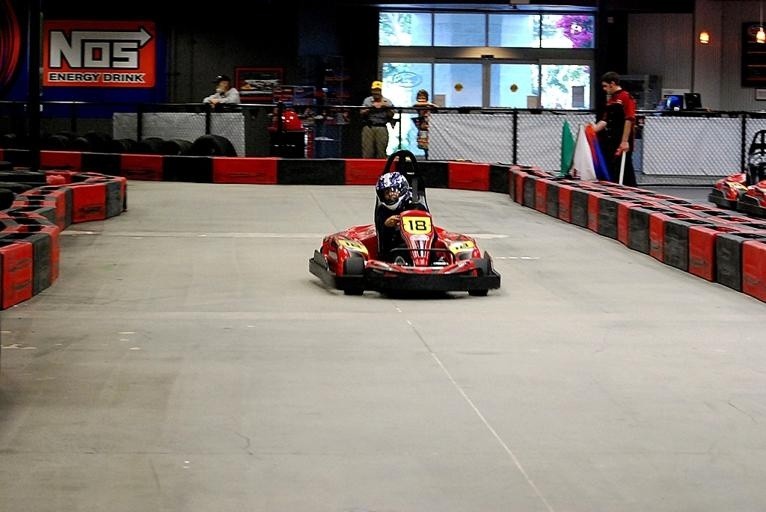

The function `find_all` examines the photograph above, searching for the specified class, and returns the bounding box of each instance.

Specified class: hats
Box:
[371,80,383,91]
[211,74,231,83]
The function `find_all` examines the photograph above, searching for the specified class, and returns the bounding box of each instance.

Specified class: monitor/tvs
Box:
[683,92,703,110]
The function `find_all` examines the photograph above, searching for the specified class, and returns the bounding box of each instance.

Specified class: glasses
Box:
[385,187,397,194]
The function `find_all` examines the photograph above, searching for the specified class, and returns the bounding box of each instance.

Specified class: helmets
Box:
[374,171,413,211]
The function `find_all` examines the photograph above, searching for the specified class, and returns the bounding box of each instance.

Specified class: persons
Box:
[374,170,451,266]
[588,71,640,189]
[202,73,241,109]
[358,80,395,160]
[409,88,441,160]
